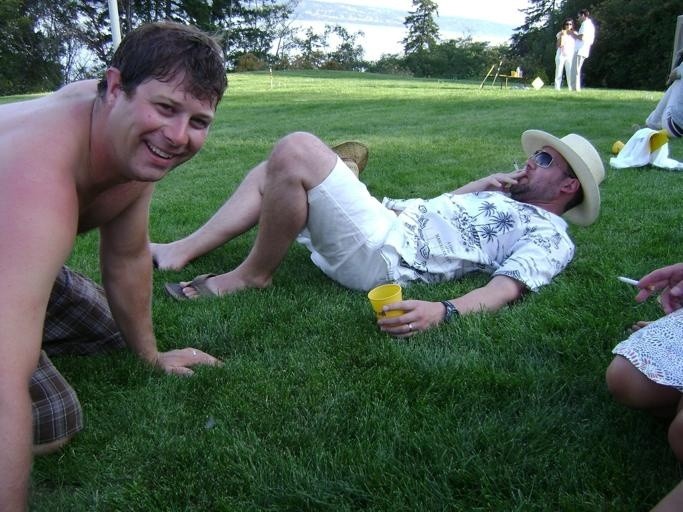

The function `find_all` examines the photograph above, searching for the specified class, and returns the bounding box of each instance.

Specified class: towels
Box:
[608,126,682,176]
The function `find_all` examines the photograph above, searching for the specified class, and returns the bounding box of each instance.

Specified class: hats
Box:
[519,128,606,228]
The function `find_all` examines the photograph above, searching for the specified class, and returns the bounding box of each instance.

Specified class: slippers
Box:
[162,271,222,303]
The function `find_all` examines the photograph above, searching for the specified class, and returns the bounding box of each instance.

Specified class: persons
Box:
[554,17,578,92]
[0,23,229,512]
[632,50,683,138]
[604,262,683,457]
[565,8,595,92]
[149,129,606,343]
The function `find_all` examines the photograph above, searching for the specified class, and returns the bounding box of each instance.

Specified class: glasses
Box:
[528,149,573,180]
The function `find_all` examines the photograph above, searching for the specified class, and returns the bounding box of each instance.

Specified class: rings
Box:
[408,323,412,332]
[192,350,197,356]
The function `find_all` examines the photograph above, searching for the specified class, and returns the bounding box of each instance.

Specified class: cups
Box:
[611,140,625,154]
[366,284,409,327]
[510,66,523,78]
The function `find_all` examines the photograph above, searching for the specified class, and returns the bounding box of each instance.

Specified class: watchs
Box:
[441,300,460,323]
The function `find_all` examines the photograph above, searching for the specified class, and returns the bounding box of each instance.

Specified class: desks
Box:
[480,73,525,89]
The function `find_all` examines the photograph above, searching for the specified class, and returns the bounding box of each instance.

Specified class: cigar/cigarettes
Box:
[616,276,655,291]
